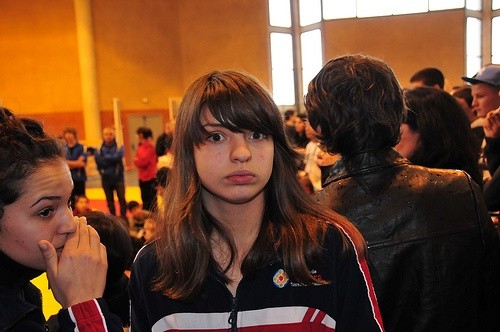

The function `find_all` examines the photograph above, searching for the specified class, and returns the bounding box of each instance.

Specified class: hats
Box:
[461,66,500,86]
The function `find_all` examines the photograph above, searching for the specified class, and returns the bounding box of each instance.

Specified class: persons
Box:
[302,54,500,332]
[63,126,173,250]
[0,106,124,332]
[394,64,500,236]
[76,211,130,328]
[129,69,385,332]
[282,109,343,197]
